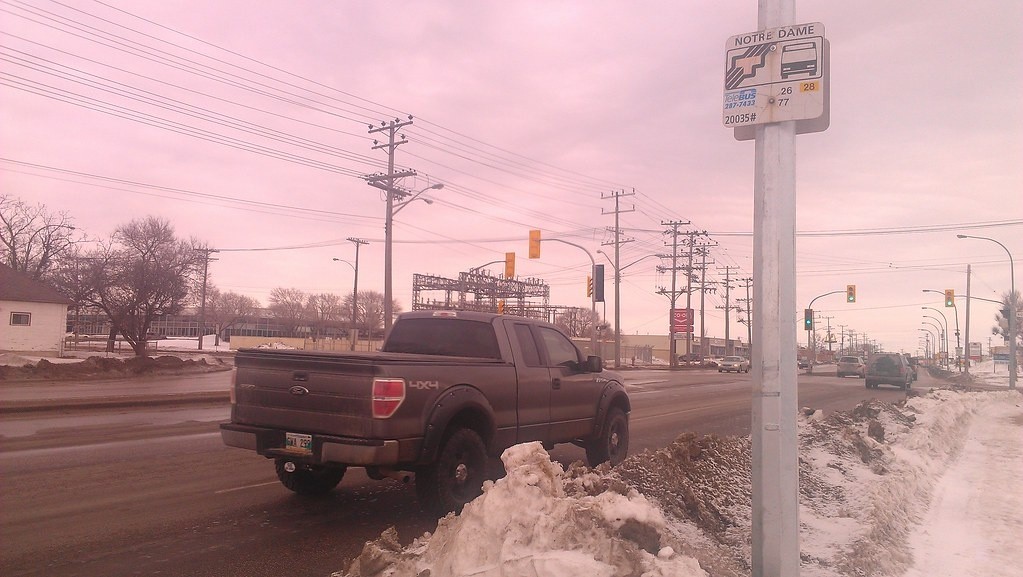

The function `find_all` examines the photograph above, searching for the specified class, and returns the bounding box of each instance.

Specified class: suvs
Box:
[864,351,913,390]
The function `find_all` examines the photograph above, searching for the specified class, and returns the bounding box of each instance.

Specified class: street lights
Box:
[922,289,961,372]
[22,222,76,274]
[923,314,945,368]
[922,322,940,366]
[957,234,1018,390]
[596,249,662,367]
[921,307,950,370]
[917,328,936,364]
[332,258,359,351]
[384,182,445,340]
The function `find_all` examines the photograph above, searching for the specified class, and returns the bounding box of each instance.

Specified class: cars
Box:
[677,352,700,362]
[799,360,813,369]
[717,356,750,373]
[907,357,918,380]
[837,355,866,378]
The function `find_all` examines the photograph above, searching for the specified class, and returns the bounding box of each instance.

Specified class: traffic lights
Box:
[586,275,593,297]
[804,309,813,331]
[846,284,856,303]
[945,290,955,308]
[498,299,505,314]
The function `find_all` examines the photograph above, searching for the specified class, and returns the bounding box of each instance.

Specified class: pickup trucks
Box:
[218,307,633,515]
